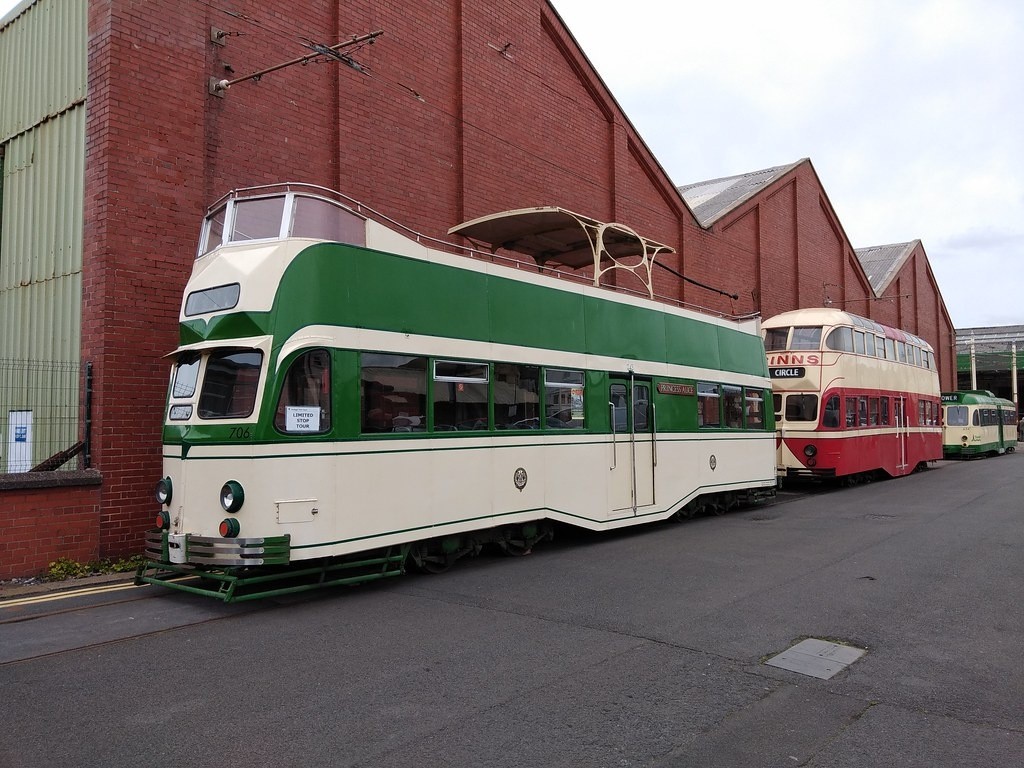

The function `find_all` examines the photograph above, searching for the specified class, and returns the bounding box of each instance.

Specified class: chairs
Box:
[392,424,413,432]
[434,424,458,431]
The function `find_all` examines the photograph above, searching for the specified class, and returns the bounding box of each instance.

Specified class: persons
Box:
[1020,417,1024,442]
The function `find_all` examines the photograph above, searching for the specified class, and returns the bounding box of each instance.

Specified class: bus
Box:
[133,181,777,607]
[941,390,1017,460]
[750,306,943,488]
